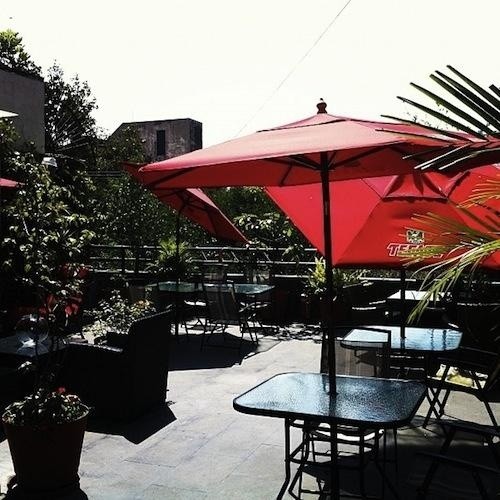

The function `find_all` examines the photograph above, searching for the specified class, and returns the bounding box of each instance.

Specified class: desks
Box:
[146,280,275,348]
[1,314,119,395]
[231,371,428,500]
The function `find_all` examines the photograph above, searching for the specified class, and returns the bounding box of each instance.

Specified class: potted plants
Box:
[1,385,89,498]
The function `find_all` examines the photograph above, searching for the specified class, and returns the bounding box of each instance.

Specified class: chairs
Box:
[66,311,168,430]
[316,281,500,499]
[124,264,271,360]
